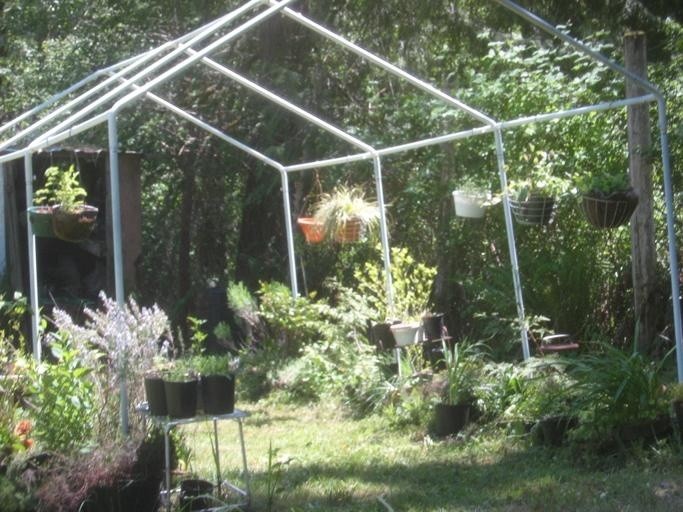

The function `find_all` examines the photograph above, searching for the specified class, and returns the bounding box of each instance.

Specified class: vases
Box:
[146,373,166,417]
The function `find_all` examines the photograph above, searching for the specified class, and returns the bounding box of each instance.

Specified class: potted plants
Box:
[519,351,676,466]
[163,323,239,419]
[27,164,100,244]
[427,335,495,438]
[296,182,379,250]
[443,160,640,229]
